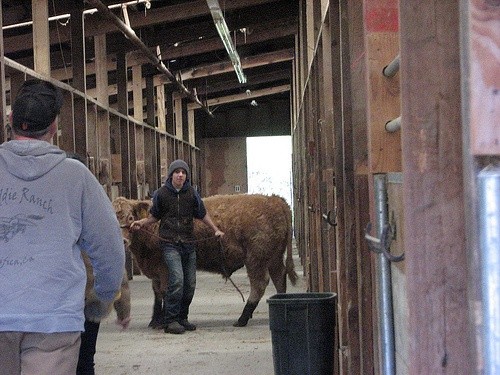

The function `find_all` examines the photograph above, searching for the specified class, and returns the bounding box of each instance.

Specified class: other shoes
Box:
[164,321,185,334]
[178,319,196,331]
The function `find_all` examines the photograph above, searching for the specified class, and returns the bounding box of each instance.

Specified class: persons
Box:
[0,79,133,375]
[130,159,225,334]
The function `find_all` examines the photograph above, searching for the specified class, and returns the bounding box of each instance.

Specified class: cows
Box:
[111,193,299,327]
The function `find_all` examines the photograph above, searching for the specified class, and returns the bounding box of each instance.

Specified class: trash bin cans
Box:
[266,292,340,375]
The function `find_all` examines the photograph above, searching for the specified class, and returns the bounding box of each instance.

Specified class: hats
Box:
[12,79,64,132]
[168,160,189,178]
